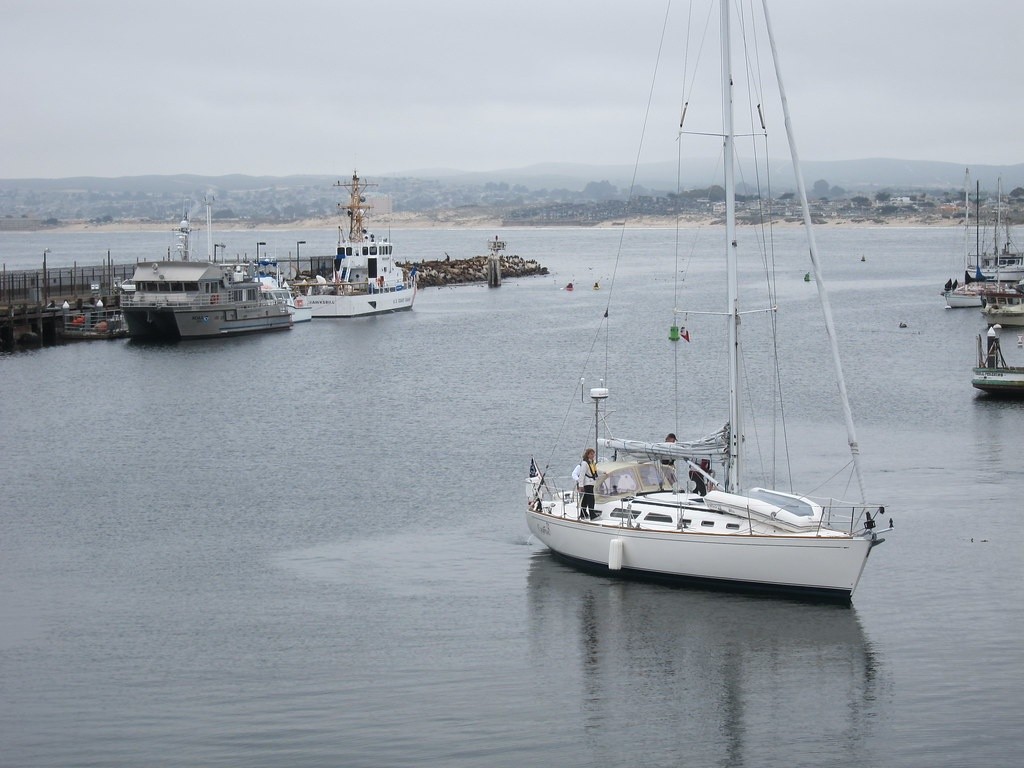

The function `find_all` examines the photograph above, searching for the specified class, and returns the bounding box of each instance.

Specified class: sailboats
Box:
[941,167,1024,328]
[524,1,896,608]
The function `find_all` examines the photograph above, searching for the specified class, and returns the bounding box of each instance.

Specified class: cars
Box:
[115,279,137,294]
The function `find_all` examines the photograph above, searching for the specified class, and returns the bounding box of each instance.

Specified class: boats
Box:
[594,286,600,290]
[566,287,573,291]
[252,263,312,323]
[971,334,1023,402]
[702,488,826,533]
[120,261,294,345]
[290,168,418,317]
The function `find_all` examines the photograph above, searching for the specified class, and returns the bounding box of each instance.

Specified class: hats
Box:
[668,433,679,441]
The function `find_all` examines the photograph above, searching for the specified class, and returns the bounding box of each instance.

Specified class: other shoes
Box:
[581,514,588,517]
[591,513,599,520]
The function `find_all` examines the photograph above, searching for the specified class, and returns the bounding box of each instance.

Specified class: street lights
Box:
[214,244,219,261]
[297,241,307,272]
[256,242,267,262]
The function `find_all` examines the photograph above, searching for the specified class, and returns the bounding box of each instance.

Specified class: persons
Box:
[658,433,685,493]
[572,450,585,499]
[578,448,602,520]
